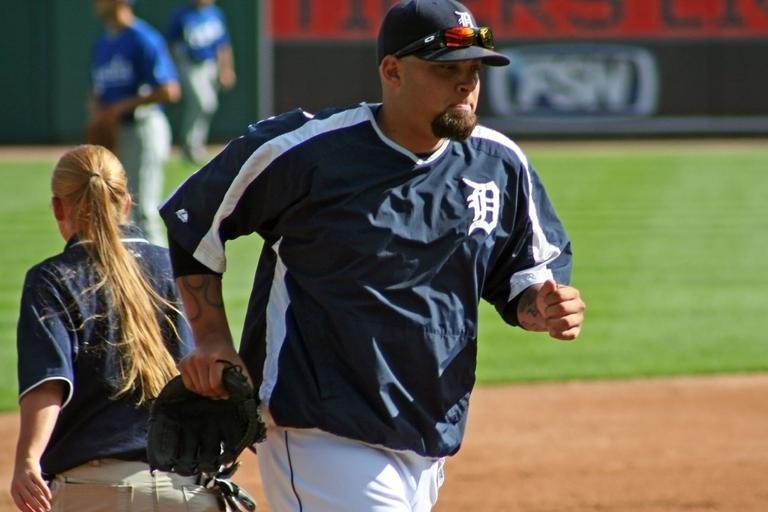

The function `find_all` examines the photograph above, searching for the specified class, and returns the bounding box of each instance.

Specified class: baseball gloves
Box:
[87,119,118,154]
[147,359,267,476]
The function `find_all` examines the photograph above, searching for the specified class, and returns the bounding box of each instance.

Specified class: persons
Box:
[8,144,228,512]
[162,1,236,168]
[159,0,589,512]
[85,1,182,247]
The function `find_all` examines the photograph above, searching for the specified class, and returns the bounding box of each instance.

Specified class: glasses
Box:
[392,26,493,59]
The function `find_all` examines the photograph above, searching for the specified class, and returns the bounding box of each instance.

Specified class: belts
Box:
[111,450,150,463]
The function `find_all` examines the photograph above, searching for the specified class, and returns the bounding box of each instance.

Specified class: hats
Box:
[377,0,511,65]
